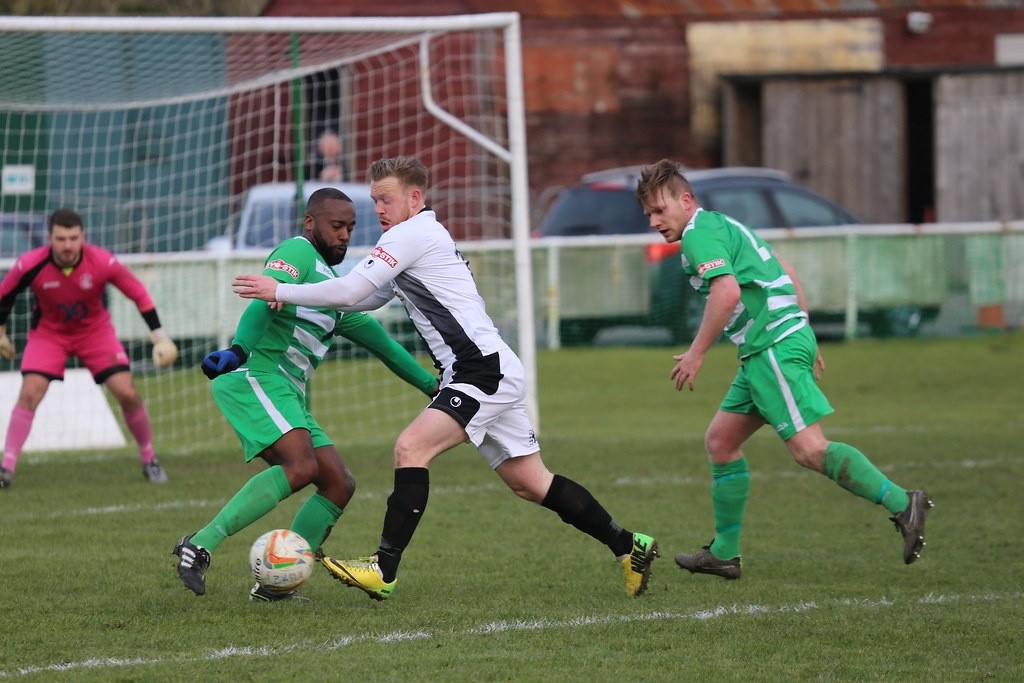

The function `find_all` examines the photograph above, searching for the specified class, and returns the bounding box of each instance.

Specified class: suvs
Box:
[529,168,941,348]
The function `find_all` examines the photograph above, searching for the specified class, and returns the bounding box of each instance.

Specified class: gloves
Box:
[148,326,179,369]
[0,324,16,359]
[200,344,248,380]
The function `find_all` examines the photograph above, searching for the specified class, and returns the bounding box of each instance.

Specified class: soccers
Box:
[248,526,315,595]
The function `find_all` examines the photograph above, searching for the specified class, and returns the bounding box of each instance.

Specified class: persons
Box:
[172,188,441,603]
[311,132,350,182]
[636,159,935,580]
[0,207,178,489]
[231,155,658,603]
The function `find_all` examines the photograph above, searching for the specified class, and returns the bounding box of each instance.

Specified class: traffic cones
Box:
[959,307,1020,336]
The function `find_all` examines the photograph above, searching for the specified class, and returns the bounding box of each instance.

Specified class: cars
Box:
[0,213,51,315]
[203,183,429,359]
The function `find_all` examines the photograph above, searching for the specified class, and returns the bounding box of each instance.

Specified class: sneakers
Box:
[142,455,167,483]
[248,580,310,604]
[0,464,10,489]
[613,532,661,599]
[673,538,742,580]
[319,554,398,602]
[171,532,211,596]
[889,488,935,564]
